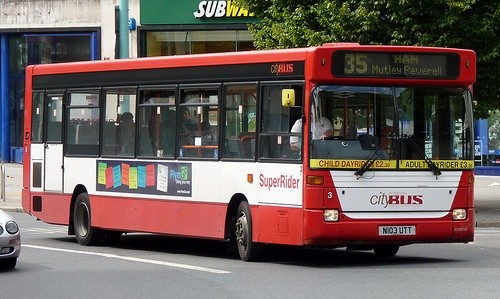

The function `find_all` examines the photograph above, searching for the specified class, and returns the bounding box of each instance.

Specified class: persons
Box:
[177,106,209,131]
[290,105,333,158]
[120,112,136,145]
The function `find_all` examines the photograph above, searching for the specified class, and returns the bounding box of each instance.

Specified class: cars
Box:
[0,208,21,271]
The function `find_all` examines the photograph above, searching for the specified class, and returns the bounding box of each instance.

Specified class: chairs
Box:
[39,121,380,159]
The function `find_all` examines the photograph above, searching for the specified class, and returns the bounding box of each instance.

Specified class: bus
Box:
[21,41,489,264]
